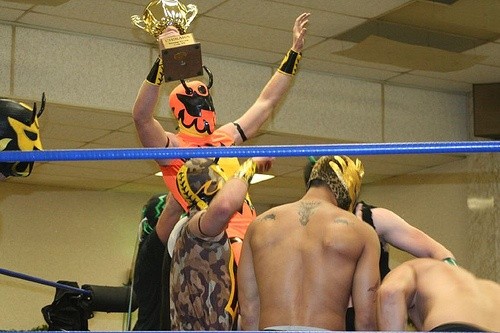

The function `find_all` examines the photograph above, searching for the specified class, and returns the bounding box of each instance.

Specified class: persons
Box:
[133,155,500,333]
[169,157,275,331]
[0,92,48,178]
[132,12,312,267]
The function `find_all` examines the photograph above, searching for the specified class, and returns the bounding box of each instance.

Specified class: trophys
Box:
[130,0,204,83]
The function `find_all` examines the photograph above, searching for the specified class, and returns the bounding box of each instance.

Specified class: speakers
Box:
[472,82,500,140]
